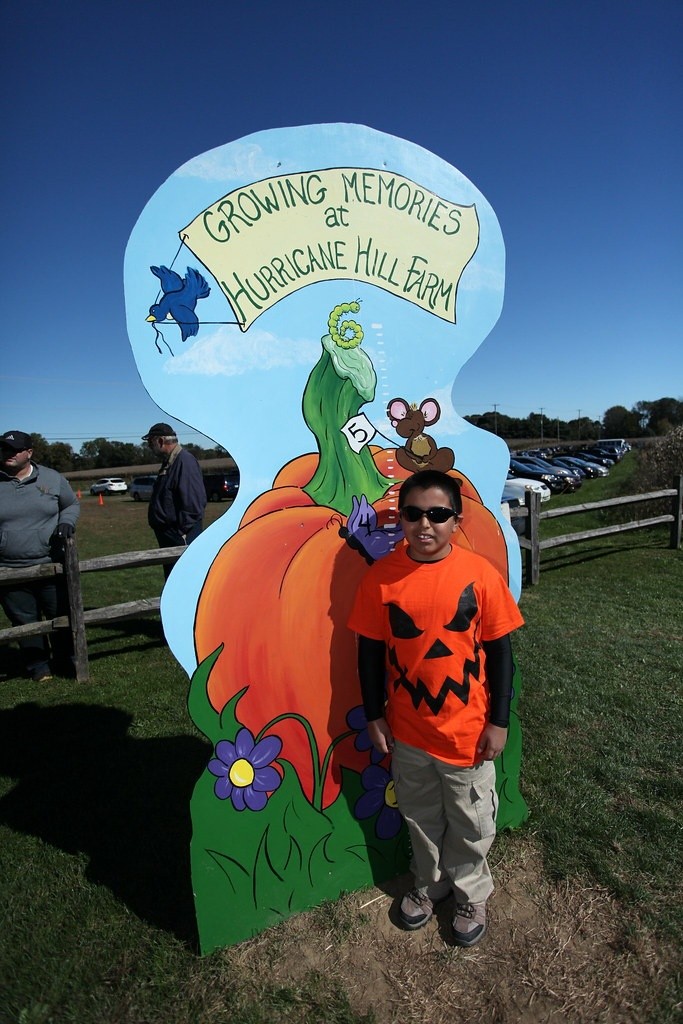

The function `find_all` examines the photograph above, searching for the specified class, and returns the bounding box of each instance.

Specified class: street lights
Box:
[578,409,581,441]
[597,415,601,440]
[492,404,499,435]
[540,407,545,449]
[557,417,560,443]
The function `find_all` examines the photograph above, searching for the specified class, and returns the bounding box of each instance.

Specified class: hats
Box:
[143,423,176,440]
[0,430,32,449]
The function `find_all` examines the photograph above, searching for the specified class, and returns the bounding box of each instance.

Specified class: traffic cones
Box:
[98,494,104,505]
[77,489,82,499]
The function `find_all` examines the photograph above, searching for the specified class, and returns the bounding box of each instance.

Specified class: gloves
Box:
[53,523,74,540]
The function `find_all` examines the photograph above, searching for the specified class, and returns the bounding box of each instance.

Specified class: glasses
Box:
[397,505,460,524]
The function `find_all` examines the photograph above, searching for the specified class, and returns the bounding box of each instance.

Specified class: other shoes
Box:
[35,672,53,681]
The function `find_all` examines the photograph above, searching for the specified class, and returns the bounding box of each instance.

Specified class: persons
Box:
[347,470,524,946]
[141,422,207,581]
[0,431,82,681]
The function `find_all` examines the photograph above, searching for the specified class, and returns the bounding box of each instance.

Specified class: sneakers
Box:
[399,884,454,930]
[450,901,487,946]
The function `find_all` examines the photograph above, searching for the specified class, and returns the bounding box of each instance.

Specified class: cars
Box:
[501,474,551,506]
[130,476,159,501]
[203,474,228,502]
[89,478,128,496]
[224,471,239,498]
[508,443,623,494]
